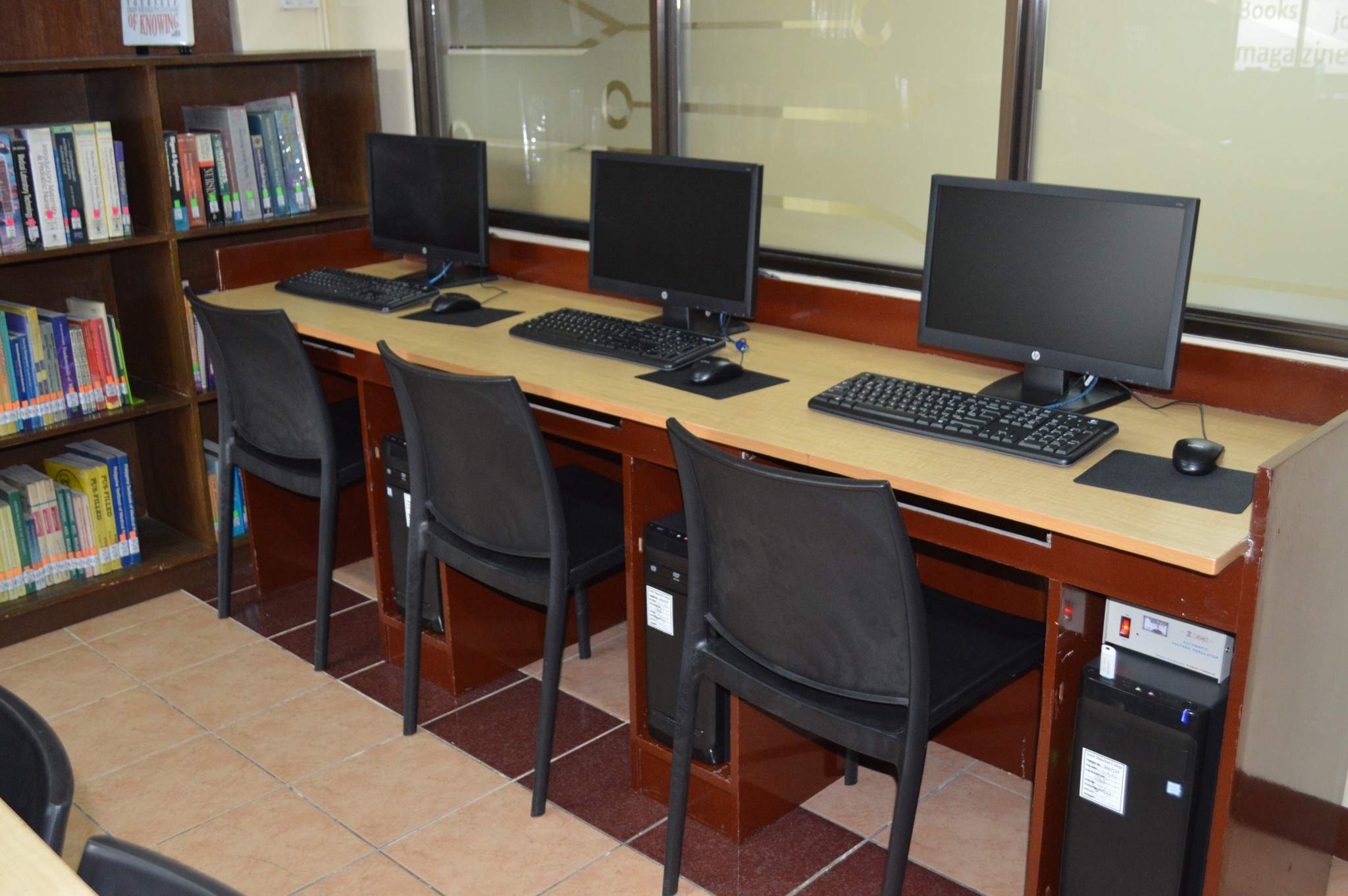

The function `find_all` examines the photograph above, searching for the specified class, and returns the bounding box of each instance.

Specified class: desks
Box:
[0,798,101,896]
[187,255,1348,896]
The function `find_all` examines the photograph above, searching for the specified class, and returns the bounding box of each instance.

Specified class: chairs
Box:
[0,683,73,866]
[662,416,1047,896]
[376,338,624,817]
[184,285,365,674]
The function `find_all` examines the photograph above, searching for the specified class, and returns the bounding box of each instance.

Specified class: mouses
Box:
[1172,438,1226,477]
[689,356,744,385]
[432,293,481,312]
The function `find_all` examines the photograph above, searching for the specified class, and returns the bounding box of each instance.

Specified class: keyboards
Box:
[274,267,440,313]
[509,308,726,371]
[808,371,1119,467]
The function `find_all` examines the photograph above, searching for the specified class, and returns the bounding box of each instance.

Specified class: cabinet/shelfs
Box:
[0,48,385,649]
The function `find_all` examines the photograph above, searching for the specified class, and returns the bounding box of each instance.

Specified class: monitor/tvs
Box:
[916,175,1201,415]
[586,150,764,337]
[365,132,499,289]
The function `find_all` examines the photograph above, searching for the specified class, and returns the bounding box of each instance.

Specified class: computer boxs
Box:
[383,430,445,636]
[1055,641,1230,896]
[642,508,731,765]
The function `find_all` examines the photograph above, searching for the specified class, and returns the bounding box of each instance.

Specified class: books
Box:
[0,121,132,255]
[163,92,316,231]
[183,279,215,392]
[0,438,142,599]
[203,436,249,542]
[0,299,134,433]
